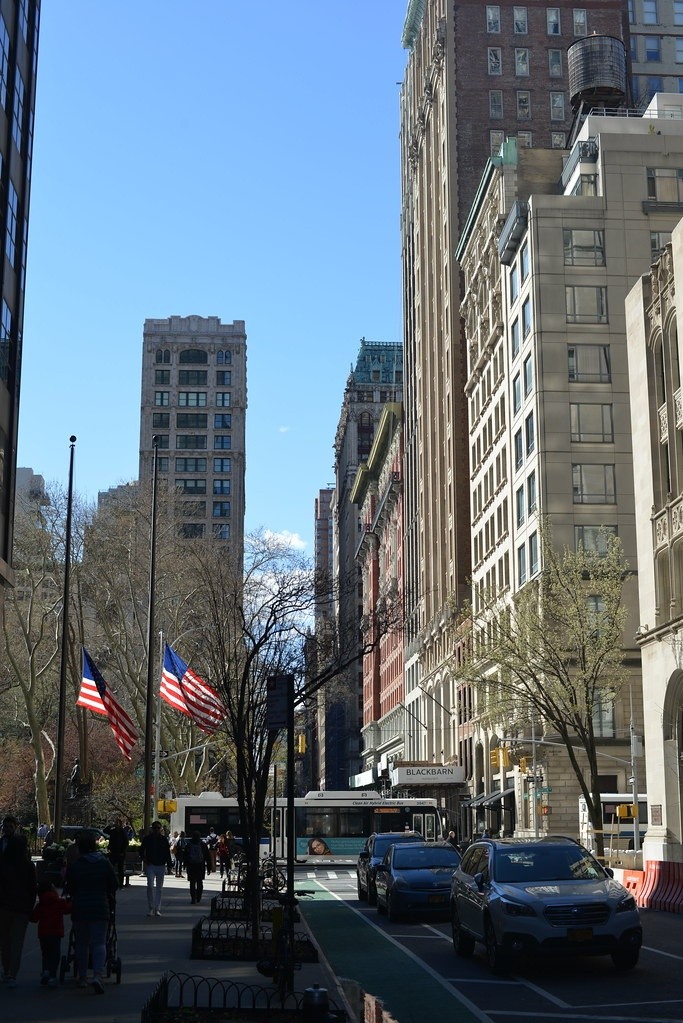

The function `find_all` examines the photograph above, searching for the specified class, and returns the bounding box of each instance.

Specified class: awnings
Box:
[462,789,514,811]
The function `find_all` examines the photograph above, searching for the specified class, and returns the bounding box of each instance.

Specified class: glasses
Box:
[152,827,161,830]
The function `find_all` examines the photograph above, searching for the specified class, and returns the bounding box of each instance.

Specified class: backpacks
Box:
[210,835,218,850]
[187,842,204,865]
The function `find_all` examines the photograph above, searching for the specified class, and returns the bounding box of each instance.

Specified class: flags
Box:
[160,641,228,735]
[76,647,139,762]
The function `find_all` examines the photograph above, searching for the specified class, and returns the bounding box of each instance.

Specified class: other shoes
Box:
[175,874,179,877]
[191,901,195,904]
[197,900,200,903]
[40,971,49,985]
[80,980,88,988]
[3,975,17,988]
[47,980,56,988]
[147,909,154,916]
[179,875,185,878]
[91,975,105,995]
[139,873,146,877]
[156,910,161,916]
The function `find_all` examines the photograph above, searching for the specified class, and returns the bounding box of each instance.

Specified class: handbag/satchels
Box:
[230,858,235,871]
[170,842,177,854]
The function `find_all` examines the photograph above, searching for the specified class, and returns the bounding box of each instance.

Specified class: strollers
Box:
[59,891,121,986]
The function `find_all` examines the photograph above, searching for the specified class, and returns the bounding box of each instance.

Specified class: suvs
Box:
[356,830,429,907]
[450,835,643,974]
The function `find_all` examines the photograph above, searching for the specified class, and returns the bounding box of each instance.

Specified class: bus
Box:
[167,791,450,871]
[578,792,647,857]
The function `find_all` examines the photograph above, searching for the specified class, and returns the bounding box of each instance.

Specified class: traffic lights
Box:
[491,747,499,767]
[519,757,526,773]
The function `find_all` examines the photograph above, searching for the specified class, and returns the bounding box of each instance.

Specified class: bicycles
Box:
[222,852,286,892]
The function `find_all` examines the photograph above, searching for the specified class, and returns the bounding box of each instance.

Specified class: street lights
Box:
[55,434,77,843]
[146,433,159,834]
[152,624,218,826]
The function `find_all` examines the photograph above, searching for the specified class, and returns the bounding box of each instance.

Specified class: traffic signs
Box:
[528,776,544,782]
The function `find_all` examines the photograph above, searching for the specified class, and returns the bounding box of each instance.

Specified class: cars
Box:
[375,840,465,922]
[41,825,110,859]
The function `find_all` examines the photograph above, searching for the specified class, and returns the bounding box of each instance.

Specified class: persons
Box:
[0,817,37,987]
[205,828,236,878]
[31,834,119,993]
[309,838,332,855]
[481,828,501,839]
[103,819,128,890]
[170,831,186,877]
[139,821,174,916]
[124,822,134,838]
[446,830,462,852]
[183,831,211,904]
[31,822,55,841]
